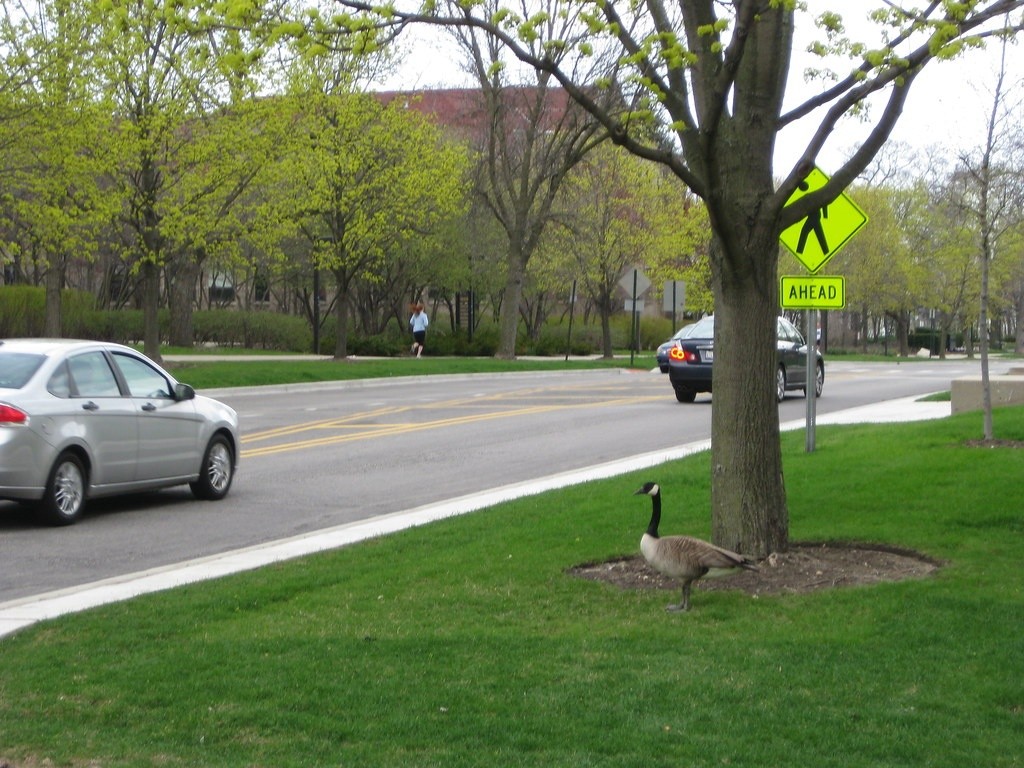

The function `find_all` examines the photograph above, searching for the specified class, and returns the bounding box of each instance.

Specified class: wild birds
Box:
[634,482,756,612]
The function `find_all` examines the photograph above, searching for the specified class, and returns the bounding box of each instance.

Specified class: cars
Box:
[0,337,243,526]
[666,316,826,403]
[657,324,698,372]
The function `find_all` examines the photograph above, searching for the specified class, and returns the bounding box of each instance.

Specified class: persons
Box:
[816,322,821,351]
[409,303,429,359]
